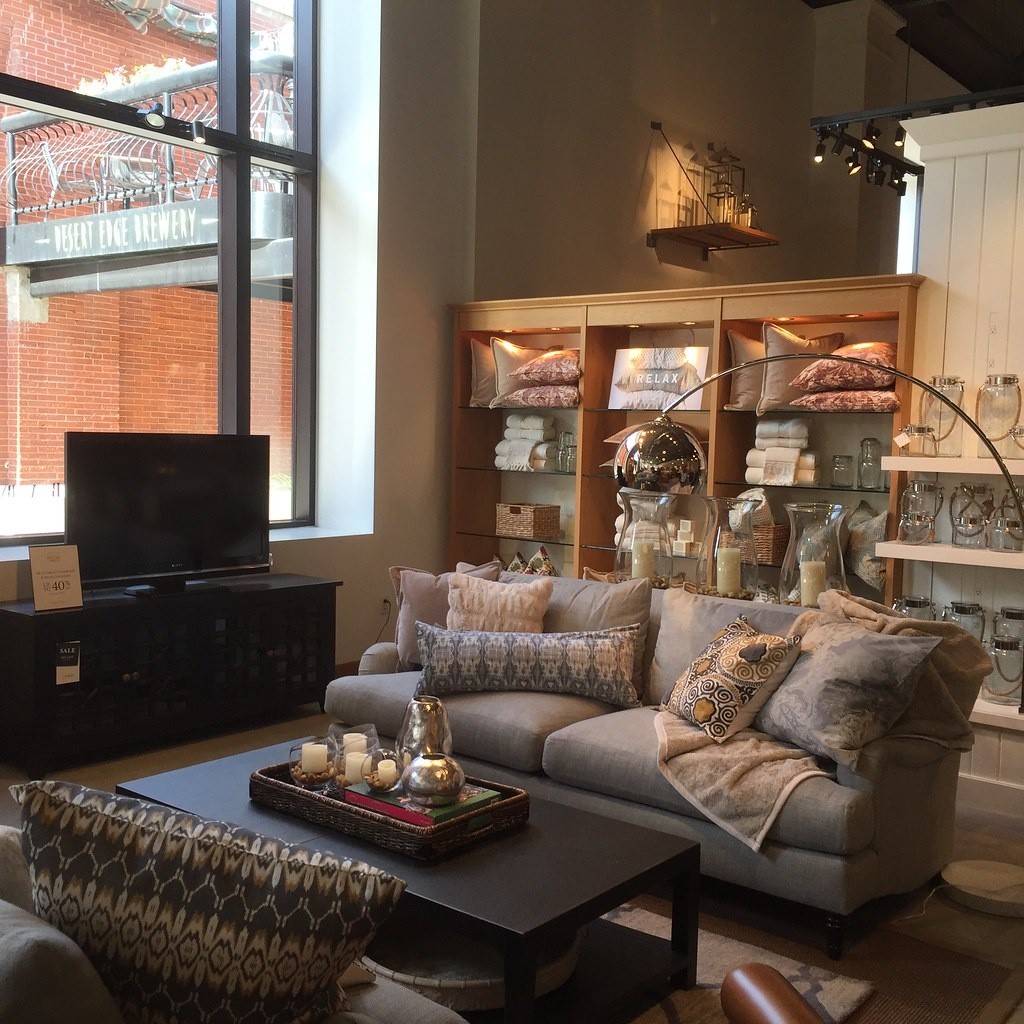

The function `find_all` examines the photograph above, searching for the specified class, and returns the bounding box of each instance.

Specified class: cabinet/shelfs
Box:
[875,455,1024,833]
[0,570,343,773]
[444,269,926,614]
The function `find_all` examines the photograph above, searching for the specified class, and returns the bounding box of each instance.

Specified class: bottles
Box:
[402,754,465,807]
[614,491,677,588]
[777,502,850,611]
[977,373,1021,459]
[898,479,1024,553]
[923,375,963,457]
[898,423,936,458]
[893,596,1024,707]
[395,696,453,769]
[695,495,762,601]
[832,437,889,489]
[565,445,577,472]
[556,432,572,472]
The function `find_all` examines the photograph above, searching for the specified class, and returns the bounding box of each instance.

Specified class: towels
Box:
[744,416,822,486]
[613,486,686,552]
[494,413,559,472]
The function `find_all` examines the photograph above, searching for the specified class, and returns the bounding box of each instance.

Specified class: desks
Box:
[92,153,161,207]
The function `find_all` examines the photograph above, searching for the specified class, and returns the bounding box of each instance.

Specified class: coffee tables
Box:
[116,735,702,1024]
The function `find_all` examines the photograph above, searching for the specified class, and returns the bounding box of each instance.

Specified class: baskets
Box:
[496,503,561,539]
[719,521,790,566]
[249,753,529,859]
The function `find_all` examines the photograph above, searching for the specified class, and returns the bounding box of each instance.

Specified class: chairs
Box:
[40,142,273,221]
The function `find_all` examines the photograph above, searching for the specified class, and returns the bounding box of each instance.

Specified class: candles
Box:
[631,535,656,577]
[302,731,395,786]
[800,556,828,608]
[717,542,741,594]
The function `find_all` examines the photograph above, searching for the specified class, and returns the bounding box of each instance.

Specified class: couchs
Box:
[325,575,994,962]
[0,825,469,1024]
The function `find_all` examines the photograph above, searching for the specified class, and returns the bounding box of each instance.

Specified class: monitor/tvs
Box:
[63,431,271,598]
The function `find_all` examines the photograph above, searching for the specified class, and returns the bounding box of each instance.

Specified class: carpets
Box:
[628,875,1024,1022]
[494,886,875,1024]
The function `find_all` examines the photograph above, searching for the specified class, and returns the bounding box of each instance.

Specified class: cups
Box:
[288,723,402,796]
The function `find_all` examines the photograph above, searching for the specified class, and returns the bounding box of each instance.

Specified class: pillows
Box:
[726,323,902,416]
[752,605,943,774]
[651,615,802,743]
[446,573,554,636]
[847,500,890,592]
[413,619,642,709]
[9,783,406,1024]
[491,546,559,577]
[469,334,581,409]
[390,562,501,666]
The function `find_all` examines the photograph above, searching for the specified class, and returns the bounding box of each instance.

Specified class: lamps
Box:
[139,103,166,130]
[810,112,908,198]
[179,123,207,143]
